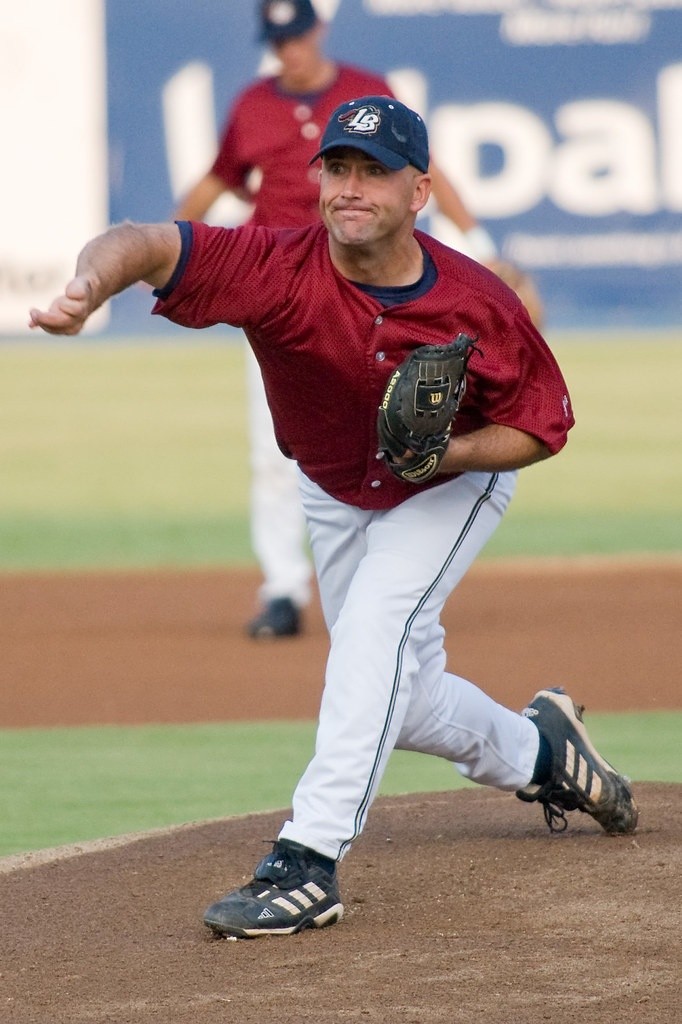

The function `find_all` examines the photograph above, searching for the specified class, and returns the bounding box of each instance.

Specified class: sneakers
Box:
[203,837,344,939]
[516,687,638,837]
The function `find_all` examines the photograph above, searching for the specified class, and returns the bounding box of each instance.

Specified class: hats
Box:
[256,0,314,41]
[308,95,429,172]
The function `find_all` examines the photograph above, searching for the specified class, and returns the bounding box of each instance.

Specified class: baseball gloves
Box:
[375,333,482,487]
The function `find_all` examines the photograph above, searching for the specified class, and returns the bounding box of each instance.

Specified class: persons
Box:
[169,0,539,636]
[30,95,639,938]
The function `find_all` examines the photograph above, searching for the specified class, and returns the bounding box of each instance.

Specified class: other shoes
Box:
[245,604,298,637]
